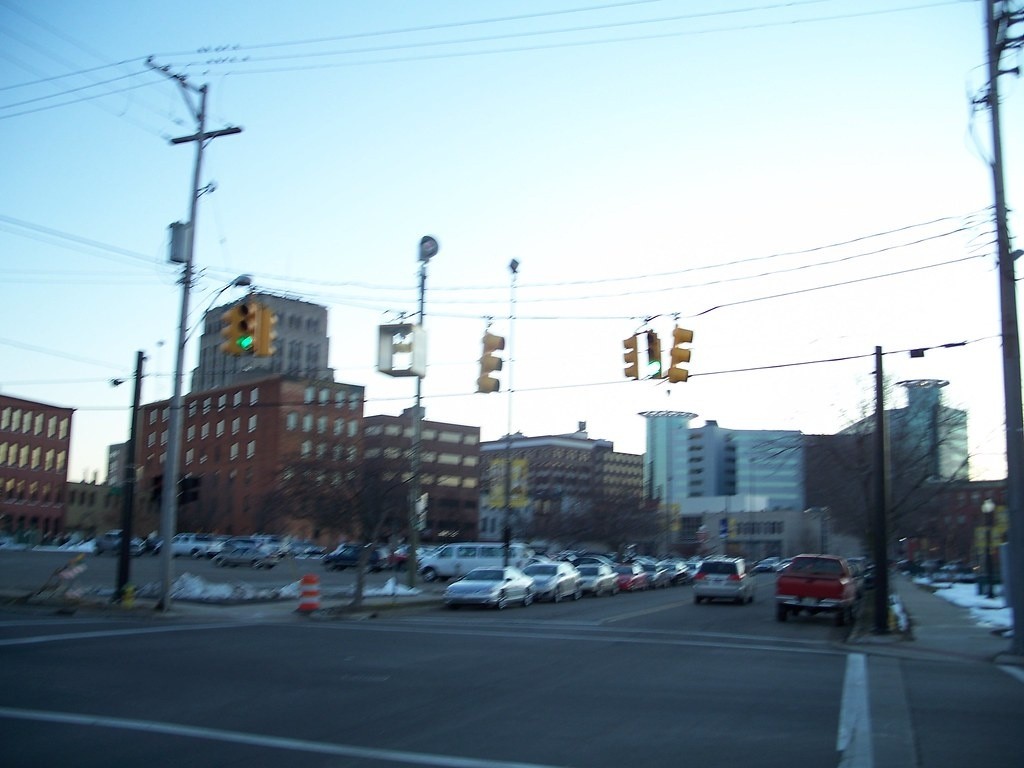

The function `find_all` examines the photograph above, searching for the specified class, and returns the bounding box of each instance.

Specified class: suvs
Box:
[847,555,878,589]
[772,553,861,627]
[692,553,759,605]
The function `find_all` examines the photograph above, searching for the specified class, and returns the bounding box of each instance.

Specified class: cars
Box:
[440,566,537,611]
[893,557,974,574]
[94,527,702,605]
[750,556,792,572]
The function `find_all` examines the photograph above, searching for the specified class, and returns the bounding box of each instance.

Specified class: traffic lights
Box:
[235,302,257,354]
[477,331,506,394]
[219,308,237,354]
[646,332,663,380]
[622,336,639,379]
[256,304,280,356]
[150,473,162,504]
[178,473,202,506]
[667,327,693,384]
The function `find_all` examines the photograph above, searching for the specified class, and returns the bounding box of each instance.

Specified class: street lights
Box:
[981,498,996,599]
[156,275,256,612]
[406,233,441,589]
